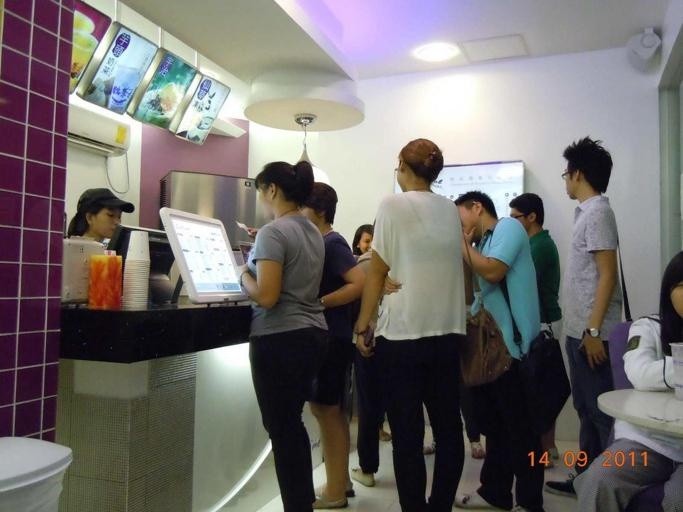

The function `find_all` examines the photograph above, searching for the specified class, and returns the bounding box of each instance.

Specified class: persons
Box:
[67,187,135,246]
[544,134,624,500]
[355,137,467,512]
[351,222,375,264]
[237,161,329,512]
[343,249,387,489]
[298,181,367,510]
[453,189,546,512]
[508,192,563,470]
[572,249,683,512]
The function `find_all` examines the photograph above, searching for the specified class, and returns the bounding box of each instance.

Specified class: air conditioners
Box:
[67,101,130,196]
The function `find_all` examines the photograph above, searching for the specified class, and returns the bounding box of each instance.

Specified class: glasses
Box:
[560,171,571,178]
[512,214,527,219]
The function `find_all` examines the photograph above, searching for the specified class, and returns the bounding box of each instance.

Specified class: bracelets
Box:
[353,325,370,335]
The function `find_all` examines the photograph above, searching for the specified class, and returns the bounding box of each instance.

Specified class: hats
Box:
[76,187,135,215]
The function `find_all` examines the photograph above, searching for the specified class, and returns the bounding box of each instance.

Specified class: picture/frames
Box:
[77,23,159,115]
[70,1,113,95]
[172,72,232,145]
[126,47,197,132]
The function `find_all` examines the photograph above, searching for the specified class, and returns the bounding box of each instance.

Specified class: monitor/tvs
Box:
[159,206,249,304]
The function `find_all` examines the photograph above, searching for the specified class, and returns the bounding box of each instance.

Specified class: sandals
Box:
[304,485,356,510]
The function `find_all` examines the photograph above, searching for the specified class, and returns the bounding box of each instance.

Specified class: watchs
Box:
[585,326,602,340]
[238,270,249,287]
[318,298,327,310]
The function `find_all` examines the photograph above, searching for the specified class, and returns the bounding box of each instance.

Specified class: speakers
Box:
[628,27,661,71]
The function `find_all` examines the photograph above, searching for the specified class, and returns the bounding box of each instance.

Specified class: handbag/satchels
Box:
[589,201,635,391]
[454,211,513,387]
[476,212,573,436]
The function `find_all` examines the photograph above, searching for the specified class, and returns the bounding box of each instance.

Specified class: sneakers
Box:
[454,488,533,512]
[421,438,437,457]
[350,465,377,486]
[466,441,486,460]
[543,448,560,467]
[546,476,579,500]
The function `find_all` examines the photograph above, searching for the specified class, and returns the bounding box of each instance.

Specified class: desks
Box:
[595,386,683,436]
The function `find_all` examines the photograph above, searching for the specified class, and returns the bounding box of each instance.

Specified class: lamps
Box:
[242,71,366,186]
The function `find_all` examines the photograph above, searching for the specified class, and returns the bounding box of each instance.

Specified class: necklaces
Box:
[276,207,301,217]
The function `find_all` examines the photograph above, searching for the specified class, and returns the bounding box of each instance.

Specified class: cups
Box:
[669,343,683,401]
[121,230,151,308]
